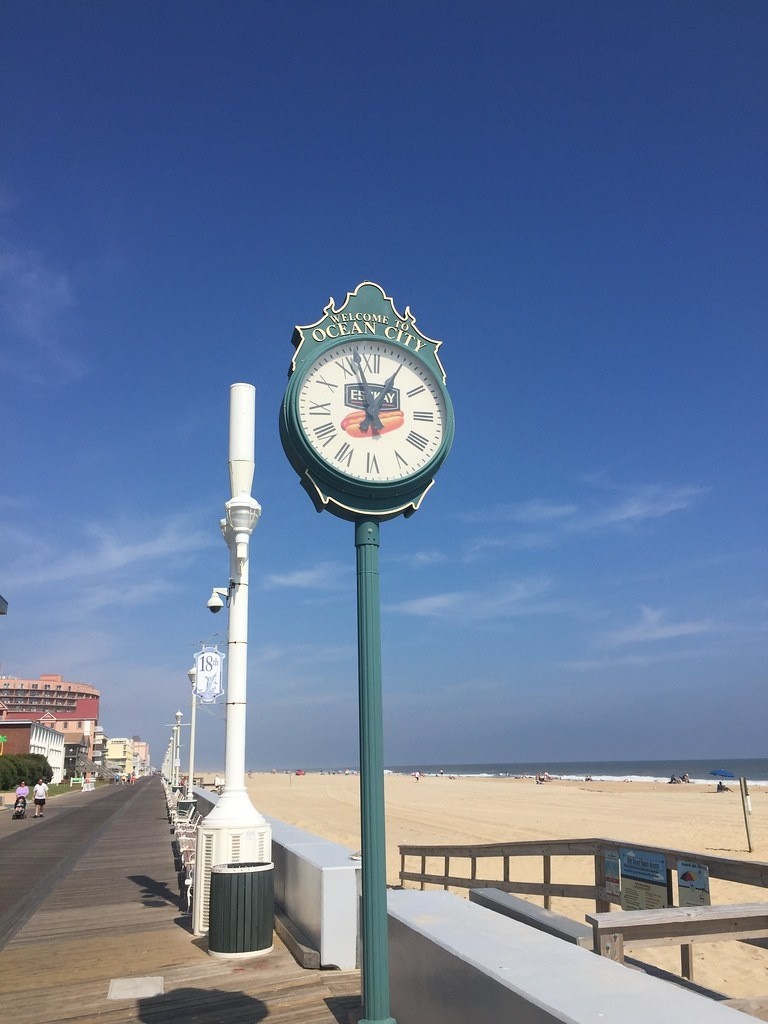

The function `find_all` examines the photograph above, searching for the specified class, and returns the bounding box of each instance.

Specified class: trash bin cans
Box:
[177,800,197,819]
[172,786,184,795]
[207,861,275,958]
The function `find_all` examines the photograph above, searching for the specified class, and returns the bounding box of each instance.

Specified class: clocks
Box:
[280,283,454,520]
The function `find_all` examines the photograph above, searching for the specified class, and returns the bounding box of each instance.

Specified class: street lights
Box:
[161,726,178,789]
[176,662,199,817]
[172,709,185,794]
[187,381,274,939]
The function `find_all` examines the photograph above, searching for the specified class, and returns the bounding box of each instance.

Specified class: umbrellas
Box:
[709,768,735,783]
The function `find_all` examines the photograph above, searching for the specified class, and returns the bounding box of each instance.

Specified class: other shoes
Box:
[40,813,43,816]
[33,815,38,818]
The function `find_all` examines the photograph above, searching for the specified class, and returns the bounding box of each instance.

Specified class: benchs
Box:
[161,779,204,914]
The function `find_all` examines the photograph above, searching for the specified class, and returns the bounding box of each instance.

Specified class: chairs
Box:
[717,784,728,793]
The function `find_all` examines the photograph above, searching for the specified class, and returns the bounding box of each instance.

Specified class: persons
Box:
[248,771,250,776]
[178,777,187,786]
[719,781,733,792]
[15,780,29,812]
[585,775,592,781]
[33,778,49,818]
[536,771,551,784]
[440,768,443,776]
[115,771,136,787]
[671,772,689,783]
[415,771,420,781]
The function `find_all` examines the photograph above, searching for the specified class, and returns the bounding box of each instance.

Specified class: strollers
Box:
[11,795,28,819]
